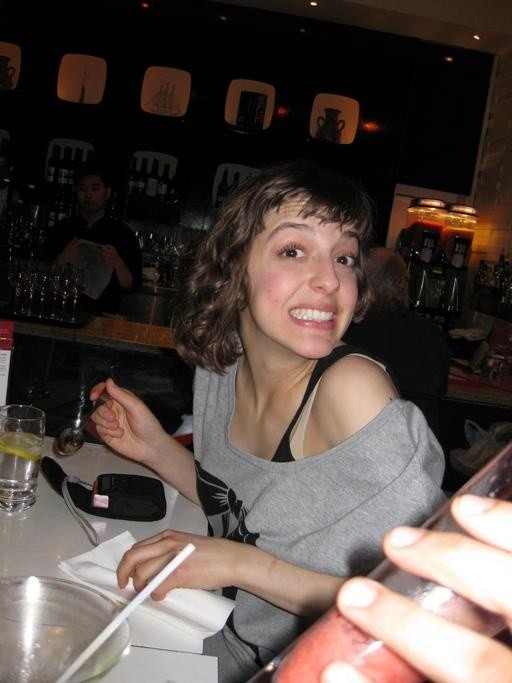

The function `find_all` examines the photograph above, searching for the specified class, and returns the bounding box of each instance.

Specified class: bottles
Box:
[0,144,92,329]
[111,153,250,288]
[0,54,16,91]
[314,107,346,142]
[473,254,512,308]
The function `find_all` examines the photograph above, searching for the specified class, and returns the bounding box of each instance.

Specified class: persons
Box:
[43,162,143,307]
[320,487,511,682]
[90,159,453,683]
[342,246,455,486]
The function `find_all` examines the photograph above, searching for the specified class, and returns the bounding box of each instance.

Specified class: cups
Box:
[249,441,512,683]
[0,404,46,514]
[234,90,268,133]
[0,574,131,682]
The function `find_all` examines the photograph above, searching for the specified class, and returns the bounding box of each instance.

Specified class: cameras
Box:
[91,473,111,508]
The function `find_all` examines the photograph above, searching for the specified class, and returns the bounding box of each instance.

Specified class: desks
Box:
[1,428,230,682]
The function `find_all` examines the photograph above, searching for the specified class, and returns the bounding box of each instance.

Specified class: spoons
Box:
[52,382,111,457]
